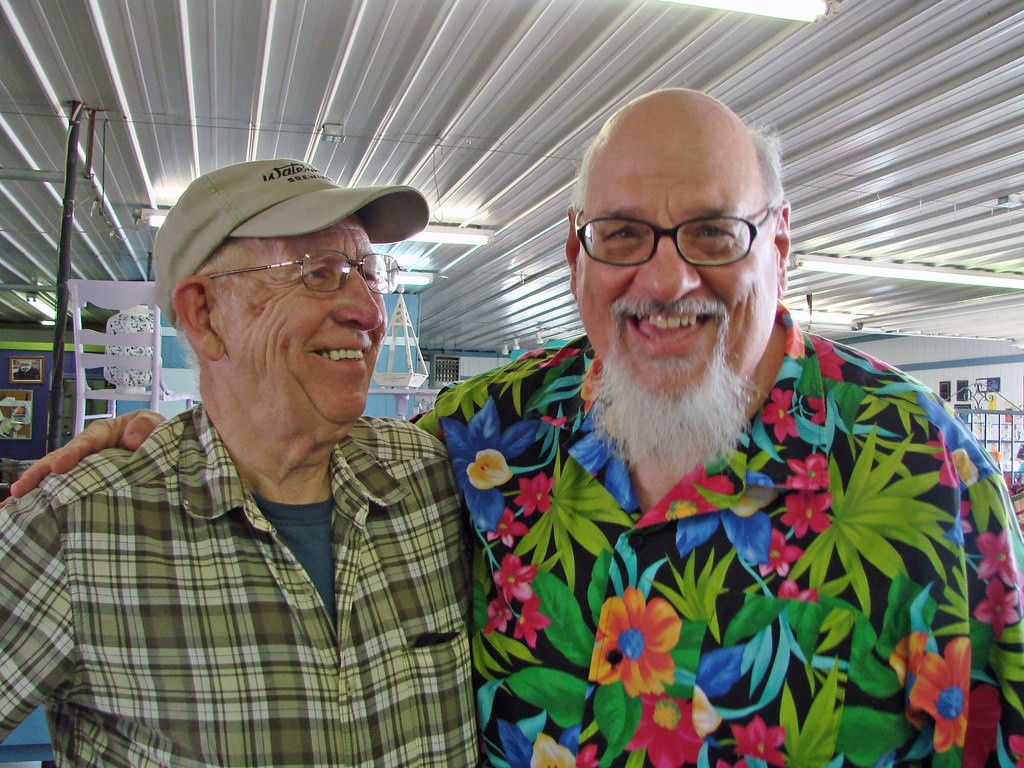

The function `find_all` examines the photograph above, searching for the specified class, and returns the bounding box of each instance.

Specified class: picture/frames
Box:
[9,356,44,384]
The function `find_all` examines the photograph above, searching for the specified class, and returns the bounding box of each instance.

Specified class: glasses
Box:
[574,207,773,268]
[207,249,403,296]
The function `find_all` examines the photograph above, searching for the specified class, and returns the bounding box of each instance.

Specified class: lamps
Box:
[512,338,520,350]
[535,332,544,345]
[789,253,1024,289]
[133,208,495,245]
[25,293,57,320]
[322,124,346,144]
[501,343,509,355]
[395,270,433,287]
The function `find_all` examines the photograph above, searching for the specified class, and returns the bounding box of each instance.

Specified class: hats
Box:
[152,160,431,329]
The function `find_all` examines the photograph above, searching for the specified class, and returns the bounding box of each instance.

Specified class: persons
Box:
[10,89,1022,767]
[1,158,484,768]
